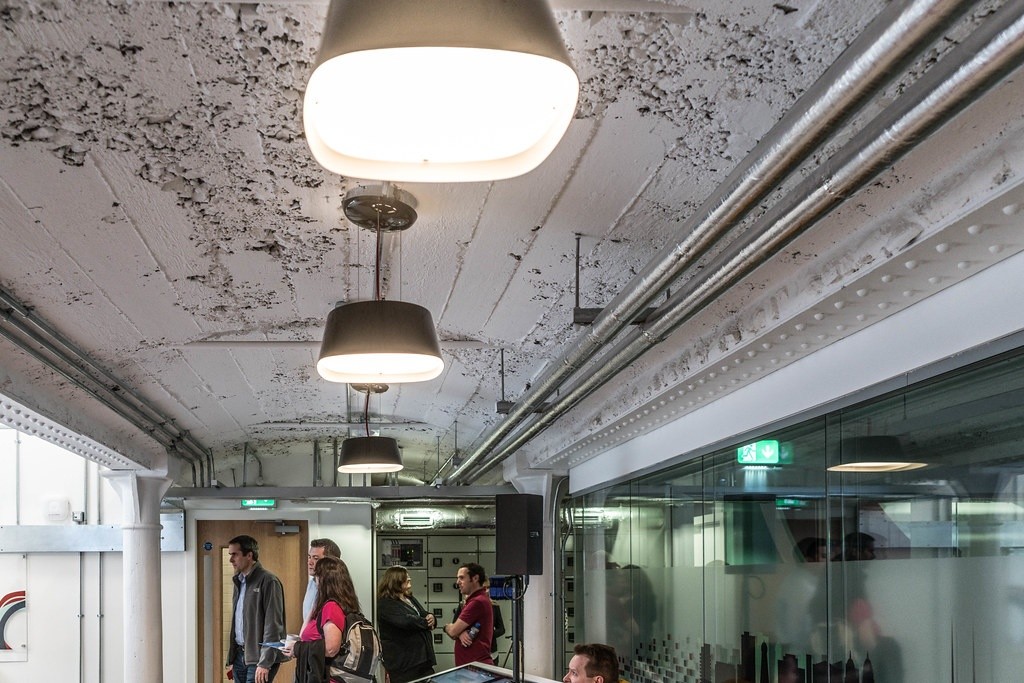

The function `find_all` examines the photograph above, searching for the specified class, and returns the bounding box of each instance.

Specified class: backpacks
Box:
[328,612,381,683]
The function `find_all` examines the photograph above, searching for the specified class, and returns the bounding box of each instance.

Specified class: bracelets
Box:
[443,624,448,631]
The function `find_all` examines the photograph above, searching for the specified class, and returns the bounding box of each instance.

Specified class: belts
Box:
[241,646,244,652]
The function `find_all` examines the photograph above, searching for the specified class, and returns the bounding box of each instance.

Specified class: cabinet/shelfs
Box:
[377,534,513,675]
[561,550,577,679]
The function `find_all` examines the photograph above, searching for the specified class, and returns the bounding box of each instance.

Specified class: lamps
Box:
[337,382,404,473]
[317,195,444,383]
[827,417,929,472]
[301,0,580,183]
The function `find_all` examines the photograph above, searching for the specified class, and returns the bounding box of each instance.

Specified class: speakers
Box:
[495,494,544,575]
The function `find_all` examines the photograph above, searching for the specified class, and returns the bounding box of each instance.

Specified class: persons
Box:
[443,564,495,666]
[281,555,360,683]
[605,562,658,670]
[301,539,342,624]
[453,575,505,667]
[377,565,437,683]
[225,535,292,683]
[771,532,905,683]
[563,643,632,683]
